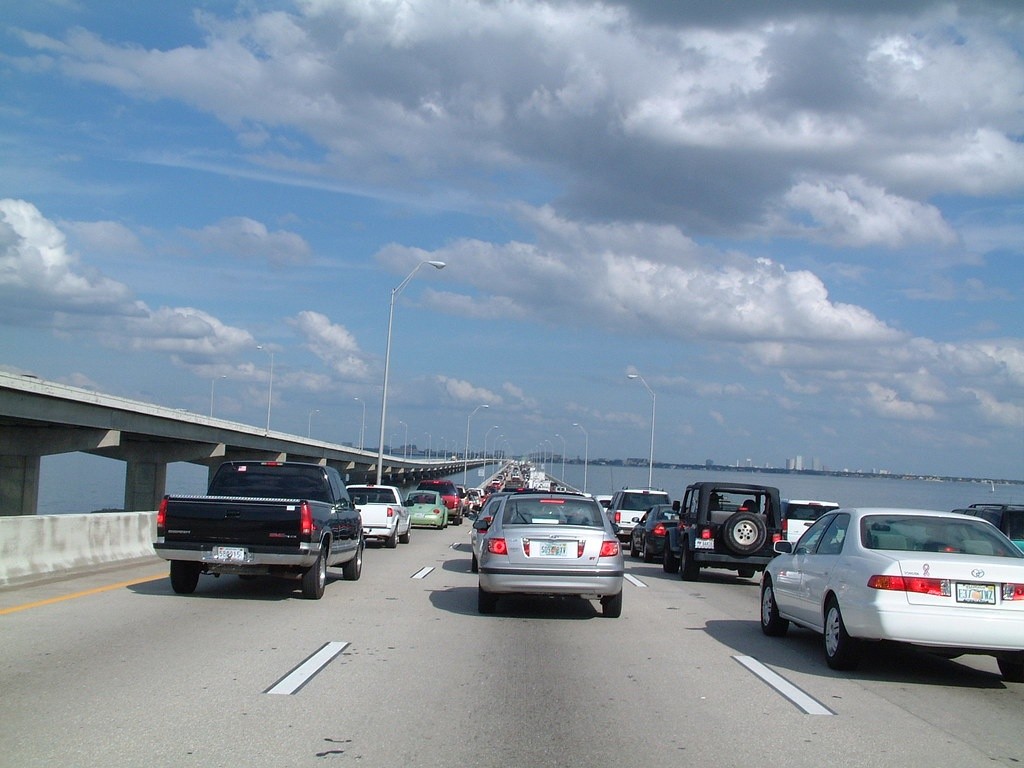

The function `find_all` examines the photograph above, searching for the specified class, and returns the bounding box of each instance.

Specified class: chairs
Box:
[512,515,530,523]
[710,502,720,511]
[959,540,992,554]
[742,500,757,513]
[875,534,907,550]
[568,514,589,524]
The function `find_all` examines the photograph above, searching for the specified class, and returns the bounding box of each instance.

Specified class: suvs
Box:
[662,481,783,582]
[762,498,839,555]
[415,479,464,526]
[605,487,672,545]
[948,503,1024,559]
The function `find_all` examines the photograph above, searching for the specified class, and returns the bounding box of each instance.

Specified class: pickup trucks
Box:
[152,460,364,601]
[345,484,412,549]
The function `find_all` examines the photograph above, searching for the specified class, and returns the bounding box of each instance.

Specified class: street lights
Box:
[307,409,320,441]
[256,345,274,434]
[573,422,589,493]
[492,434,507,475]
[627,372,656,488]
[484,425,499,482]
[529,433,566,481]
[210,374,227,416]
[375,260,447,484]
[353,397,366,450]
[462,404,489,486]
[398,420,458,461]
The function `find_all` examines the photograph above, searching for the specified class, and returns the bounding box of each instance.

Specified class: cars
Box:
[405,490,449,530]
[592,494,615,514]
[470,491,522,576]
[759,507,1024,683]
[453,460,592,518]
[629,504,692,564]
[473,489,624,619]
